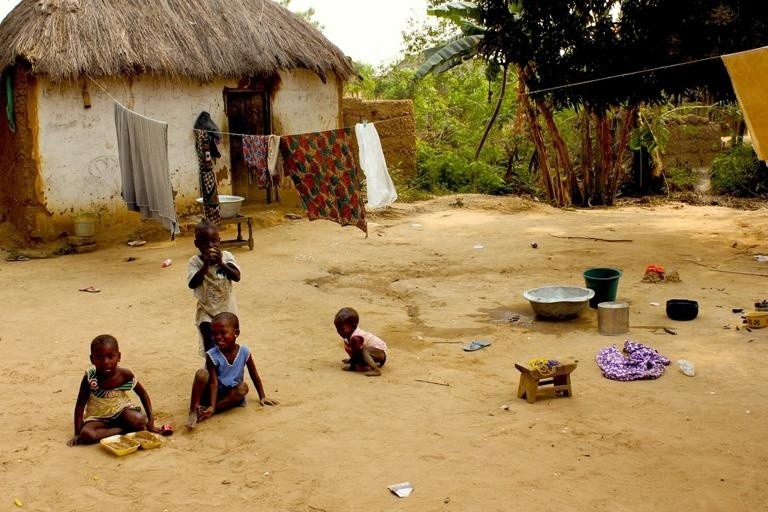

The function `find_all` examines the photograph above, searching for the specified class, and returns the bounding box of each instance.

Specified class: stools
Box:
[200,215,253,249]
[515,361,577,403]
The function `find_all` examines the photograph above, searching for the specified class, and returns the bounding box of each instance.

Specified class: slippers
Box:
[6,256,30,261]
[464,340,491,351]
[79,286,101,293]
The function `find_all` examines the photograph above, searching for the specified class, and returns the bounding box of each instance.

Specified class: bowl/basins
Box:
[523,286,596,320]
[195,195,245,218]
[666,299,698,321]
[745,311,768,328]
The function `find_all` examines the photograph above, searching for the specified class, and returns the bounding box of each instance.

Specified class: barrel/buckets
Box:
[598,300,628,336]
[583,268,623,310]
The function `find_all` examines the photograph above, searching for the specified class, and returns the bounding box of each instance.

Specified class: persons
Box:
[334,307,388,376]
[185,312,280,429]
[67,334,162,447]
[188,221,240,353]
